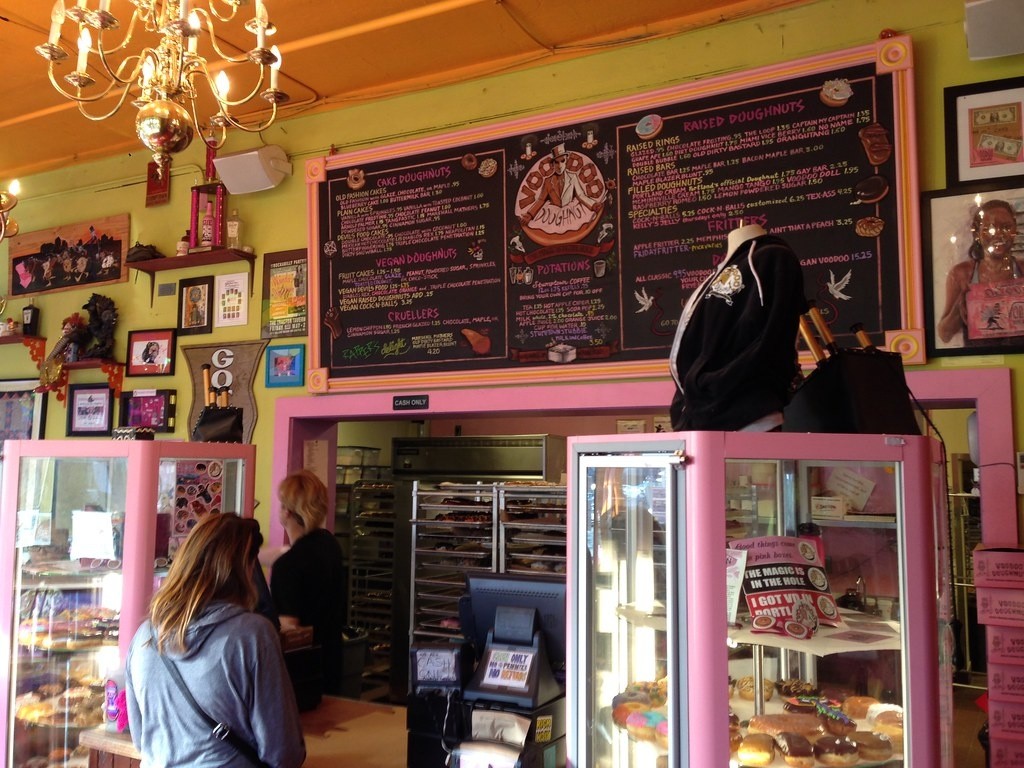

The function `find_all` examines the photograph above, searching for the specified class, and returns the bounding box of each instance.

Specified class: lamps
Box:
[35,0,289,180]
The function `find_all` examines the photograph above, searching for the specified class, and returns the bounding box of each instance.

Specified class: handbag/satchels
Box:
[192,405,243,443]
[781,347,923,434]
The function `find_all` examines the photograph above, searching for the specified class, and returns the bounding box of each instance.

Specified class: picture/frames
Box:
[68,249,307,436]
[919,76,1024,357]
[0,378,49,460]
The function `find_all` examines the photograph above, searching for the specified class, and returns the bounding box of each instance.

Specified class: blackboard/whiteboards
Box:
[304,36,927,396]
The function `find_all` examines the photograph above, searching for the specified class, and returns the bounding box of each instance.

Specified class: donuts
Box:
[611,675,670,752]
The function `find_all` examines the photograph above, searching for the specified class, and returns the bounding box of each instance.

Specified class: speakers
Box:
[212,144,288,195]
[963,0,1024,61]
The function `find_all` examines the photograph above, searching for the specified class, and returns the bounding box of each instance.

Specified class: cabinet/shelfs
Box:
[0,438,259,768]
[567,428,940,767]
[349,480,567,645]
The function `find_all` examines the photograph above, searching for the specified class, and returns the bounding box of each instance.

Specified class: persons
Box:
[938,199,1024,347]
[268,470,349,710]
[124,511,306,768]
[668,223,814,430]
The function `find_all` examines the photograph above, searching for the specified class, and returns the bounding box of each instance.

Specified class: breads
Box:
[21,744,90,768]
[354,483,395,652]
[13,679,110,728]
[18,605,119,650]
[441,616,462,629]
[433,481,566,575]
[726,672,908,768]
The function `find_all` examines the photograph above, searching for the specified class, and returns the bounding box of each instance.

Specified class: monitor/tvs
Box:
[464,576,567,710]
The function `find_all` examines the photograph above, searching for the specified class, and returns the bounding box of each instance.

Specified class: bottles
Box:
[23,298,39,336]
[226,209,244,251]
[202,201,216,247]
[182,230,191,241]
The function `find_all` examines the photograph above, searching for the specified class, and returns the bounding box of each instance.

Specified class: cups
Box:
[176,242,189,255]
